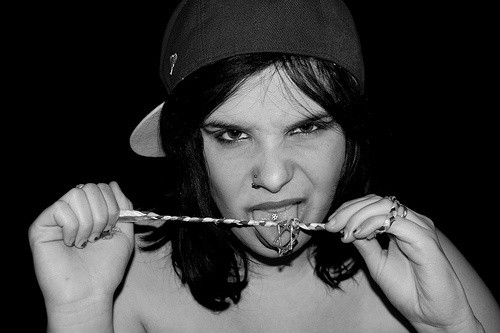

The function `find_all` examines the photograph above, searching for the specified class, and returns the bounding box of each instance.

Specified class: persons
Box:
[28,1,499,333]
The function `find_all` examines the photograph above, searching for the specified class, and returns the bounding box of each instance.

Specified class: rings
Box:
[401,206,408,219]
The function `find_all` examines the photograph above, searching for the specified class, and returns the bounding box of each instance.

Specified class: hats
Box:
[130,0,365,158]
